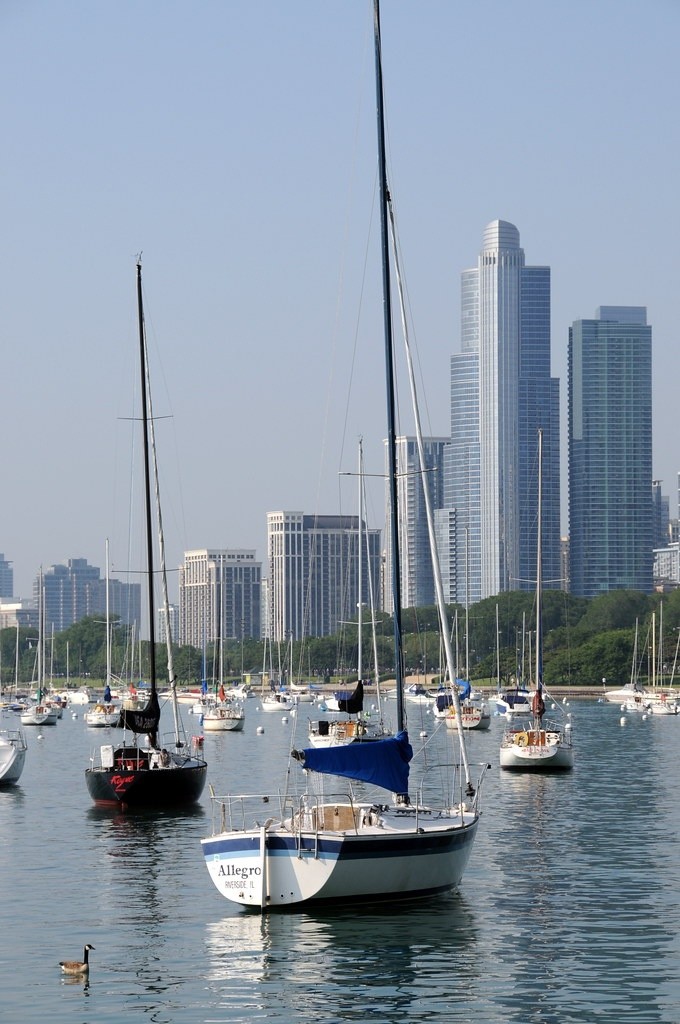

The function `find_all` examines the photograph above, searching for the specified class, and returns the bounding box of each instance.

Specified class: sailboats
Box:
[85,536,119,725]
[202,553,244,730]
[84,253,207,807]
[70,607,152,704]
[445,524,491,730]
[500,427,574,768]
[0,588,71,718]
[202,1,490,907]
[261,596,350,711]
[206,913,480,978]
[388,600,482,703]
[20,563,58,725]
[602,601,680,715]
[487,611,549,719]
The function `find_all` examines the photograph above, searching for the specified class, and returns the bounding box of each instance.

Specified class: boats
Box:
[0,728,27,783]
[159,684,257,714]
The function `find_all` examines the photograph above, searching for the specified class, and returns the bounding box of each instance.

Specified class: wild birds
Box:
[59,944,96,973]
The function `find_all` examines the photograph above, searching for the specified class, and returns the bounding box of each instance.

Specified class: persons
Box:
[363,679,372,685]
